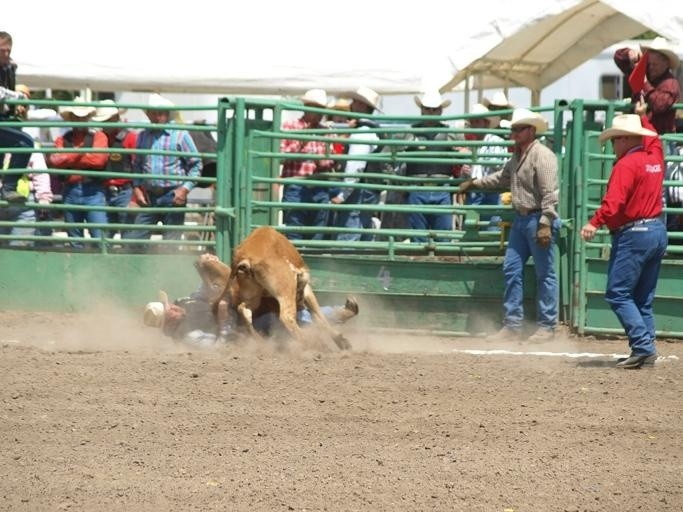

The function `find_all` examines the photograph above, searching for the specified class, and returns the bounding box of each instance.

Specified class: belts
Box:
[519,208,540,216]
[109,183,130,197]
[609,216,658,234]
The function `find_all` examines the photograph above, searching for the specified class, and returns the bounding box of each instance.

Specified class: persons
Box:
[278,87,567,255]
[667,117,683,255]
[457,108,563,343]
[144,286,358,352]
[0,31,216,254]
[580,94,667,370]
[615,37,681,258]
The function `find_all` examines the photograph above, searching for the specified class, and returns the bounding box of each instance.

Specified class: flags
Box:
[627,49,649,96]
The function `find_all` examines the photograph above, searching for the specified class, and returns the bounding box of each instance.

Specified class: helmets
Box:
[16,84,31,99]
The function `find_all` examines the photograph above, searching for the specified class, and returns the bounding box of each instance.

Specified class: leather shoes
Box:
[486,328,521,342]
[529,328,554,344]
[345,295,358,319]
[616,352,658,368]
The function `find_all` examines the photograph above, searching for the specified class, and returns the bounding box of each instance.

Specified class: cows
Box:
[193,225,352,351]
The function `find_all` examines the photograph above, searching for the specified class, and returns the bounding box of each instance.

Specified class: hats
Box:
[142,290,169,338]
[414,89,451,108]
[465,91,548,135]
[143,99,179,120]
[89,99,127,122]
[598,113,658,141]
[640,36,681,70]
[296,86,383,114]
[59,97,97,122]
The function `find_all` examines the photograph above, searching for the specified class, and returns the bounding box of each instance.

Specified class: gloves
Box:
[456,177,478,194]
[536,224,552,249]
